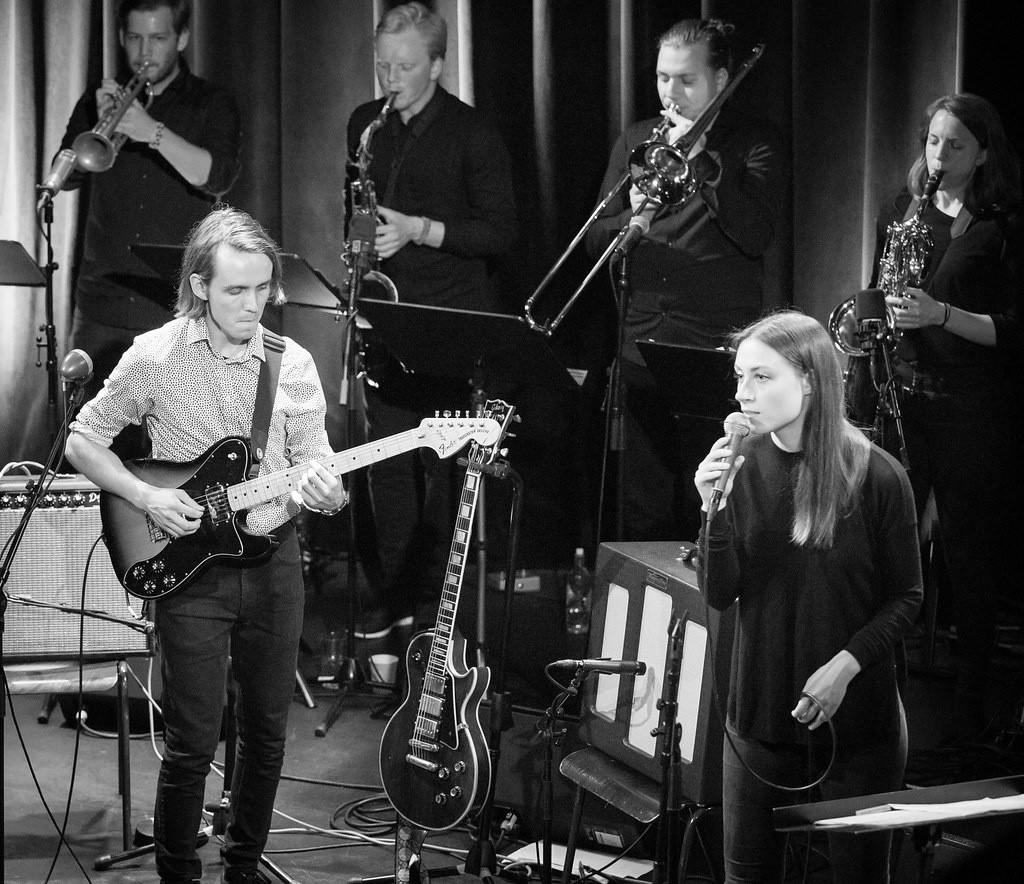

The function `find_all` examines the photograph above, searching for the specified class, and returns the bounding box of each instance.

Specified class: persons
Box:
[585,19,777,541]
[51,0,244,460]
[64,207,345,884]
[344,0,520,638]
[694,312,924,884]
[867,93,1024,695]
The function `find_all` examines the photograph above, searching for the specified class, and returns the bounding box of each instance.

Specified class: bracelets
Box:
[942,302,951,327]
[149,122,164,147]
[414,217,431,246]
[323,491,347,516]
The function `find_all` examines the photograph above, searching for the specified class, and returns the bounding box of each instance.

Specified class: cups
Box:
[367,655,399,696]
[319,629,350,689]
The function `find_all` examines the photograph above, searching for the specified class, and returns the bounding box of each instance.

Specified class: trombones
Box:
[522,38,768,337]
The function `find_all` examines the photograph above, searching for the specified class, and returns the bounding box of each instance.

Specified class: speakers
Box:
[478,699,655,858]
[579,541,738,807]
[0,472,154,663]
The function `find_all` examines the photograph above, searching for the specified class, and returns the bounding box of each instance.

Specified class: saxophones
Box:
[338,90,401,331]
[827,168,946,401]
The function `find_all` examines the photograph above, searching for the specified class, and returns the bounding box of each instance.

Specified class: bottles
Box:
[565,548,593,634]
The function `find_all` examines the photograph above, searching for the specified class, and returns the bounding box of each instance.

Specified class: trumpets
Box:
[73,58,155,173]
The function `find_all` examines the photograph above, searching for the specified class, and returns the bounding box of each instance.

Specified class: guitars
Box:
[378,398,524,832]
[100,406,504,603]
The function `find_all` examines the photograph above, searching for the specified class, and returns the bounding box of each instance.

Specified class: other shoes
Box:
[346,595,414,639]
[916,630,1000,676]
[221,867,272,884]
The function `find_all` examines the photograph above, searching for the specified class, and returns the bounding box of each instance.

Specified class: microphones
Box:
[348,214,375,277]
[555,659,646,676]
[37,149,78,209]
[856,288,886,364]
[706,412,751,522]
[611,215,650,263]
[61,349,93,380]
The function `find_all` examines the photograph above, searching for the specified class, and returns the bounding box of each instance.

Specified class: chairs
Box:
[560,746,724,884]
[0,658,133,851]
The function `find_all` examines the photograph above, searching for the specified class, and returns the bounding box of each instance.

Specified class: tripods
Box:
[348,455,534,884]
[292,264,399,737]
